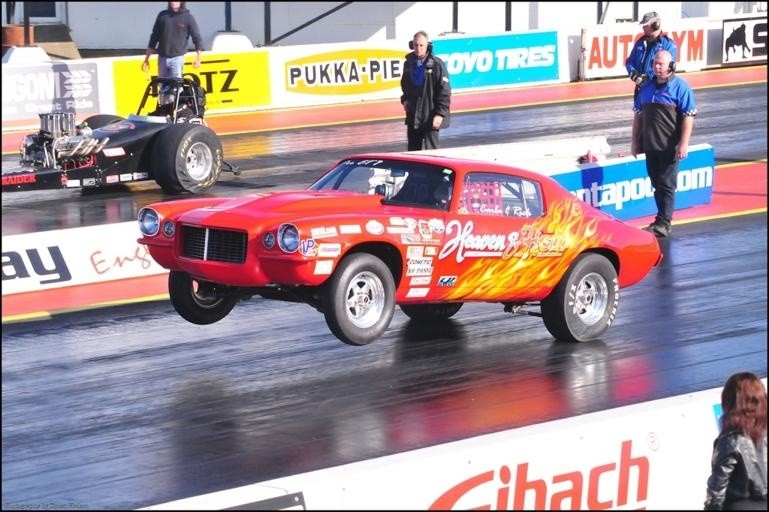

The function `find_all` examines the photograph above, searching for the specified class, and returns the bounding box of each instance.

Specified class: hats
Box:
[640,12,660,25]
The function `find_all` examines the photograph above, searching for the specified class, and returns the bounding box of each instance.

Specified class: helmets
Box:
[158,83,188,112]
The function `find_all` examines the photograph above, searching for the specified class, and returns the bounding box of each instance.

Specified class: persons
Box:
[626,9,678,153]
[699,367,768,512]
[141,0,206,107]
[399,30,452,152]
[631,48,697,242]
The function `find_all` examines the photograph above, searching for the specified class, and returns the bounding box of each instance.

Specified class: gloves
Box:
[630,70,649,87]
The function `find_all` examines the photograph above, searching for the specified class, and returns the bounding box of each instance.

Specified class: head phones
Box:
[652,18,661,31]
[668,58,677,74]
[409,40,432,53]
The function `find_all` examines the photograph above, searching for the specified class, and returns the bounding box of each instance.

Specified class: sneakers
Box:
[643,224,672,237]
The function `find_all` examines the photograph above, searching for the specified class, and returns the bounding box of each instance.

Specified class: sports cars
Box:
[134,151,660,349]
